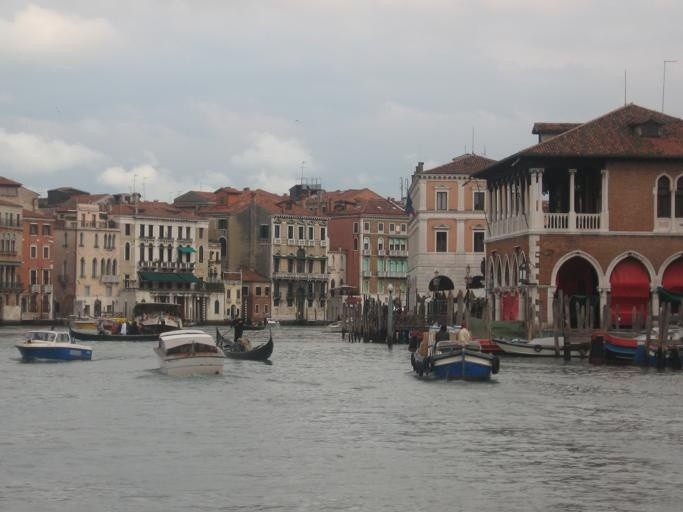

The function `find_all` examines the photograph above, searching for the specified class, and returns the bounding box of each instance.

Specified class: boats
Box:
[66,314,183,343]
[151,328,227,377]
[14,328,95,363]
[327,315,354,333]
[595,324,683,365]
[492,333,591,358]
[213,326,276,361]
[407,334,501,382]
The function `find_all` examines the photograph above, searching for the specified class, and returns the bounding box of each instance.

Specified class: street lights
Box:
[385,283,396,349]
[432,268,440,299]
[465,264,471,312]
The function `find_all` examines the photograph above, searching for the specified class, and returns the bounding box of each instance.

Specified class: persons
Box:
[96,311,166,336]
[230,315,245,343]
[434,324,449,354]
[457,322,470,344]
